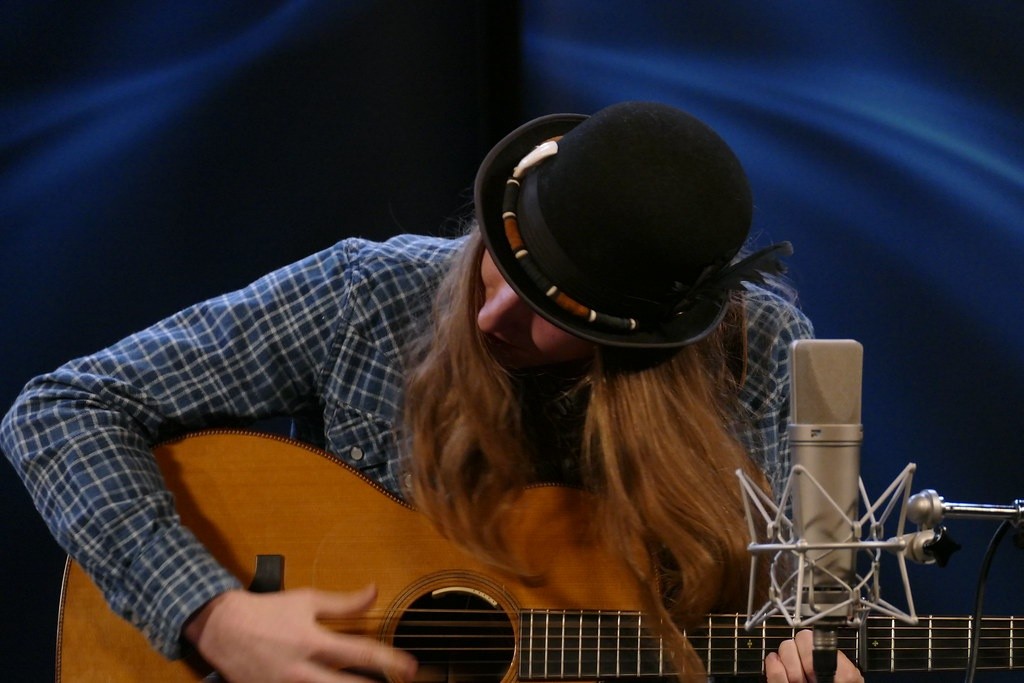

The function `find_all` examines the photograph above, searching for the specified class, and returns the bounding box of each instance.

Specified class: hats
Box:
[473,100,795,350]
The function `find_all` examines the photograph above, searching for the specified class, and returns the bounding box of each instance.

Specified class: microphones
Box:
[786,337,863,683]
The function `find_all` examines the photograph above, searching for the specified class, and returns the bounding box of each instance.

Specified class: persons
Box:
[0,102,865,683]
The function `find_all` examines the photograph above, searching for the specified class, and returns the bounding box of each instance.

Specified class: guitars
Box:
[53,429,1024,683]
